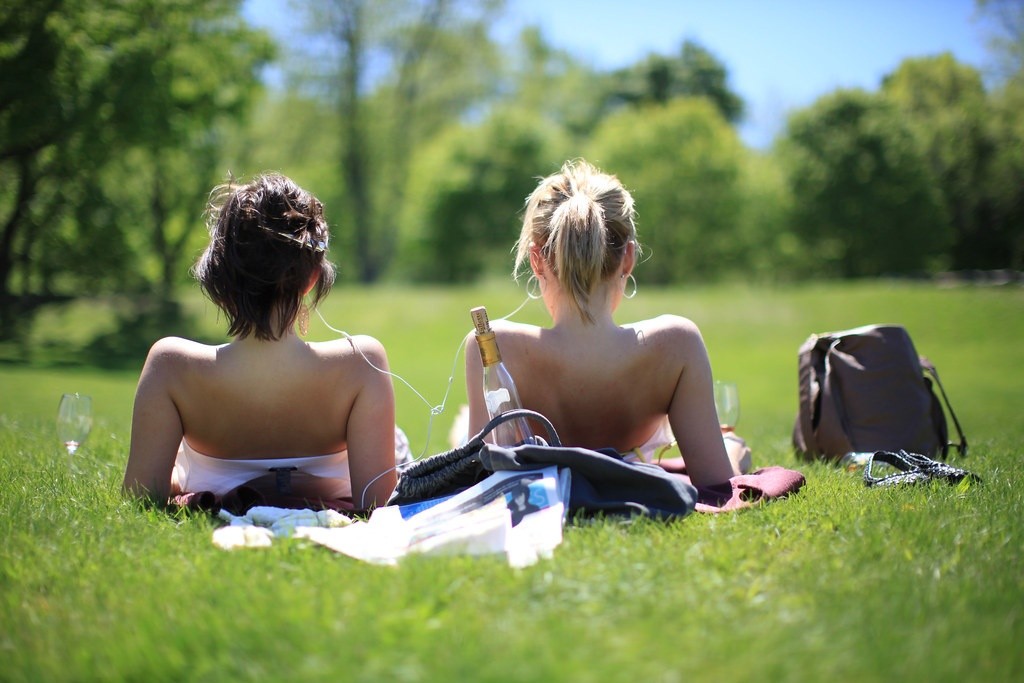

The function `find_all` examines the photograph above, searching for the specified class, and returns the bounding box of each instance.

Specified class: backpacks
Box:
[793,319,968,466]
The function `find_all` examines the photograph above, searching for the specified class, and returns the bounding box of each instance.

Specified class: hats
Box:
[507,485,531,508]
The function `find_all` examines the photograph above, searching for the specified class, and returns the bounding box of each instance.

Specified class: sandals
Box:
[862,448,929,489]
[900,449,985,486]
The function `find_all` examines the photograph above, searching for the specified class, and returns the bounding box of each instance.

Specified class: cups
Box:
[56,392,95,456]
[711,380,740,436]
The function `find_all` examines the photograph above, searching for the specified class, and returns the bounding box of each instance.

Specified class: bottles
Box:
[471,306,533,450]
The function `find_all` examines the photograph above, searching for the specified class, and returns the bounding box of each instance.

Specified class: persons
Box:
[508,486,540,526]
[121,174,397,512]
[465,162,753,488]
